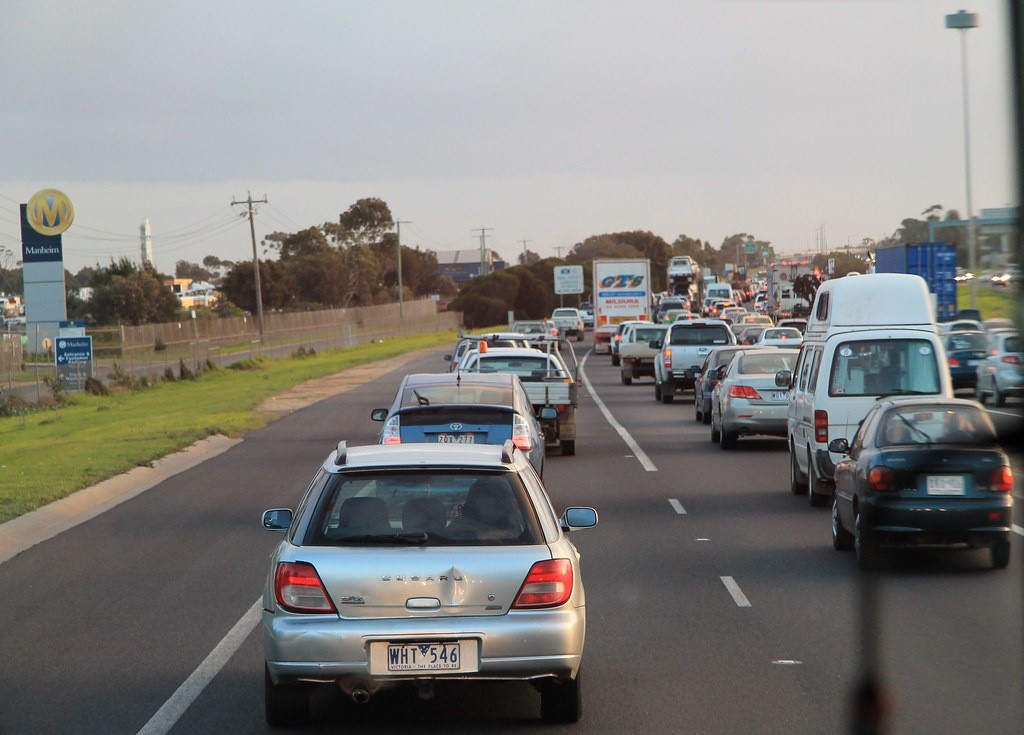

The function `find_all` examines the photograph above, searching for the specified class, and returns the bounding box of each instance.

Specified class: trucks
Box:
[592,258,653,353]
[873,243,956,318]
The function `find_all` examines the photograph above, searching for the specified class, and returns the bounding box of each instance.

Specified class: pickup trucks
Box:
[453,331,583,455]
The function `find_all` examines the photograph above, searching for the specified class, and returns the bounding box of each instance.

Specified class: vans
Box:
[774,272,955,509]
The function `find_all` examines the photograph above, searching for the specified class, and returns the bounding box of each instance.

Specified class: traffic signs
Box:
[54,336,94,390]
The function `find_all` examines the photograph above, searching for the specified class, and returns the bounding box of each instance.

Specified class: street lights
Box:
[946,10,981,309]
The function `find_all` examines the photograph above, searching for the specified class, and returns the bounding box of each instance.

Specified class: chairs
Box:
[341,494,391,531]
[400,497,448,537]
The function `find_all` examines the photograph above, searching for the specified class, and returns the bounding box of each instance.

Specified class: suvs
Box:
[261,439,600,727]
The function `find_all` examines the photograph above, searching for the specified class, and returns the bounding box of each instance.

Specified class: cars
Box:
[444,307,594,372]
[611,256,1024,449]
[371,372,558,480]
[828,396,1014,571]
